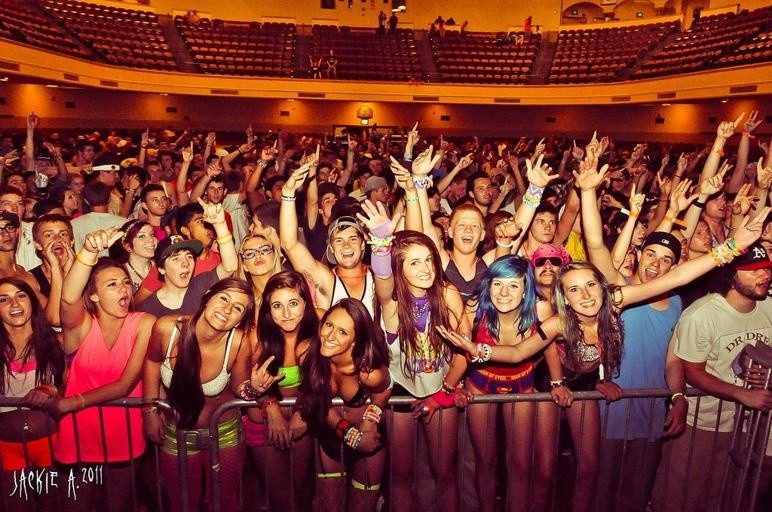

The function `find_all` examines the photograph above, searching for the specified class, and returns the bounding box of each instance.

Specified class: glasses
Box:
[0,223,16,234]
[242,245,272,259]
[534,256,563,267]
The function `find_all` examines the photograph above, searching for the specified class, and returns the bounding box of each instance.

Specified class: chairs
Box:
[0,0,772,84]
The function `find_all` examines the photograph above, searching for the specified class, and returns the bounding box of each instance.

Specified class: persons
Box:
[428,15,468,37]
[184,10,193,18]
[327,50,338,78]
[389,12,398,30]
[494,15,541,46]
[577,13,586,23]
[378,11,386,29]
[309,47,322,78]
[0,112,771,510]
[190,10,208,31]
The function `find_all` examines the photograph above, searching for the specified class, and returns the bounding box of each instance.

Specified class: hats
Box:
[155,234,202,265]
[92,151,120,171]
[733,243,772,270]
[360,176,386,198]
[640,232,680,264]
[326,216,365,264]
